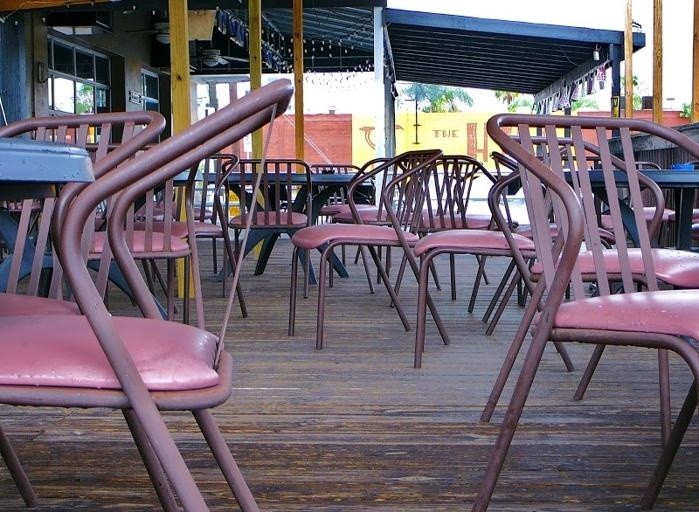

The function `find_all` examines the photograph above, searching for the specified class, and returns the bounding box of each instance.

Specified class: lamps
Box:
[205,61,218,66]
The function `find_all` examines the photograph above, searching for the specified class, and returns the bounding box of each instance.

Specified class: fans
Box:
[191,40,250,65]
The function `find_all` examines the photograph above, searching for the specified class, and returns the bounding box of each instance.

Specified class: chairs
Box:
[0,78,699,510]
